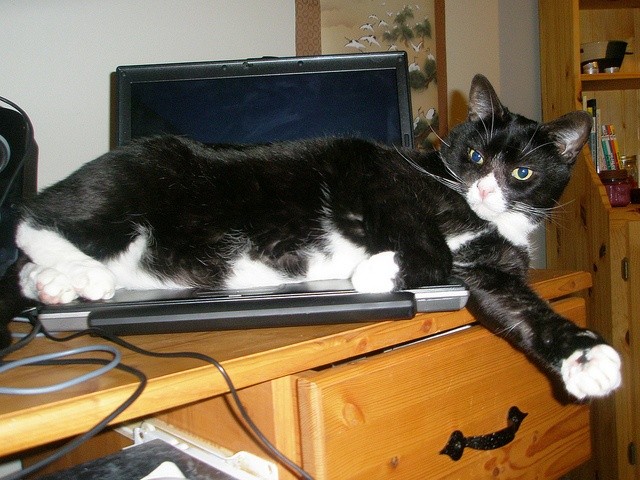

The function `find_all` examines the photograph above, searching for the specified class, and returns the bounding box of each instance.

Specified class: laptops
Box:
[37,50,418,337]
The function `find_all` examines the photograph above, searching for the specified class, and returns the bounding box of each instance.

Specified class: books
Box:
[583,95,621,173]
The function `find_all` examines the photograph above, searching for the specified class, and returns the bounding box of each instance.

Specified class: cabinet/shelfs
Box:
[538,1,640,479]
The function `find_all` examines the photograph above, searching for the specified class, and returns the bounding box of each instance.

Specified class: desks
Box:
[0,272,597,480]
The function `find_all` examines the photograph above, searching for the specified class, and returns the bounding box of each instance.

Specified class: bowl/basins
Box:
[580,40,628,75]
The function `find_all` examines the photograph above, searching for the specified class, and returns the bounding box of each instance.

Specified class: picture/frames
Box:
[295,1,448,155]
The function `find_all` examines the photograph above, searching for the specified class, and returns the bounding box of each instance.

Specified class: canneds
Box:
[597,168,631,208]
[617,154,638,193]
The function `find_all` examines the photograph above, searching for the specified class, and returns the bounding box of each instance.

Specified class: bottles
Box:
[620,154,637,183]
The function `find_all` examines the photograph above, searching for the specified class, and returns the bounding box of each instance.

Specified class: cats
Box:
[0,70,625,403]
